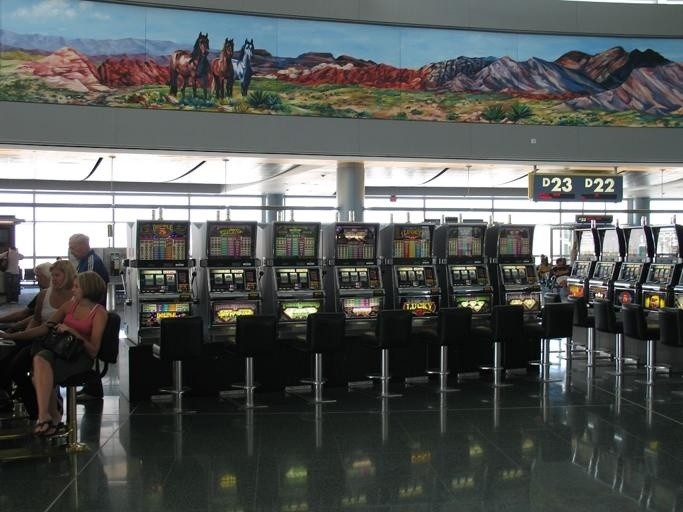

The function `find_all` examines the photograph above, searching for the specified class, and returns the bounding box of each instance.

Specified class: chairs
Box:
[216,315,277,410]
[471,306,525,388]
[412,307,472,393]
[362,310,412,398]
[48,311,121,454]
[288,311,346,404]
[523,302,576,378]
[152,315,204,416]
[542,293,683,384]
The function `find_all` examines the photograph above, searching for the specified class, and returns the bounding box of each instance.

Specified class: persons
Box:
[69,234,109,402]
[0,246,24,302]
[0,271,108,437]
[57,257,62,261]
[8,260,77,421]
[0,262,52,411]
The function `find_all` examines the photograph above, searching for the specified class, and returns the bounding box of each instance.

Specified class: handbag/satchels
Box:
[39,320,84,361]
[0,250,10,272]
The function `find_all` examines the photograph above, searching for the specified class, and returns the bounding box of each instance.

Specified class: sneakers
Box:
[74,390,103,401]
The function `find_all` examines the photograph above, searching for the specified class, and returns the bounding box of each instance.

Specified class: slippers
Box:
[33,416,62,437]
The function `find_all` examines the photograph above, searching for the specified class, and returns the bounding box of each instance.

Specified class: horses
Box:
[230,38,254,96]
[208,37,234,100]
[168,32,208,99]
